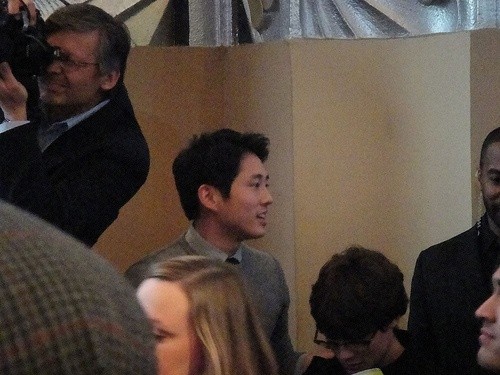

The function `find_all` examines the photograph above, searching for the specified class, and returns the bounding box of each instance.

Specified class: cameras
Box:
[0,0,56,99]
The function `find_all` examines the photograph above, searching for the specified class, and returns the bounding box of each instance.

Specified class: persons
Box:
[122,126,312,375]
[297,243,415,375]
[0,0,151,249]
[473,262,500,370]
[0,198,159,375]
[132,253,279,375]
[408,127,500,375]
